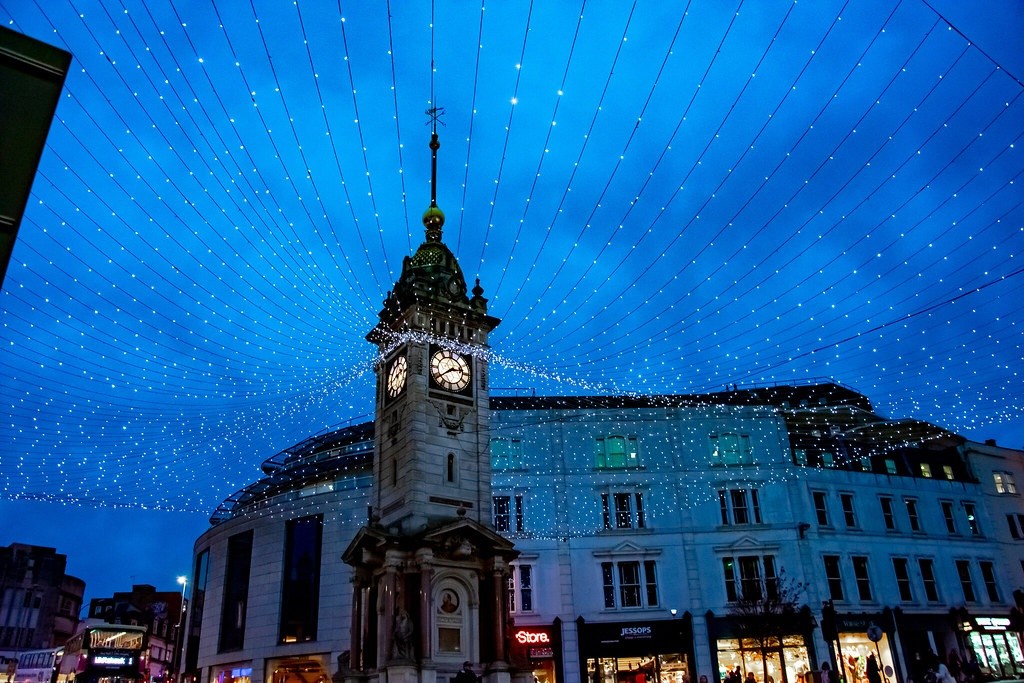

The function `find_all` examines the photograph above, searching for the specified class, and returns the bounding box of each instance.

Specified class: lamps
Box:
[670,609,678,618]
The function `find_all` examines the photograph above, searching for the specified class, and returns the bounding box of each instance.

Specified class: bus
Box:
[56,624,149,683]
[13,645,66,683]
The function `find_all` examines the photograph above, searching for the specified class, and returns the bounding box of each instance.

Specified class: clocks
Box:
[387,355,408,400]
[430,350,471,392]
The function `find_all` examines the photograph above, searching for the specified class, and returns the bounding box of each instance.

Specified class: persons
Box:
[745,672,757,683]
[867,655,882,683]
[820,662,837,683]
[725,646,964,683]
[395,607,413,658]
[455,661,482,683]
[700,675,707,683]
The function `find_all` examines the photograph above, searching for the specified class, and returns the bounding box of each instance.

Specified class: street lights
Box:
[173,575,186,673]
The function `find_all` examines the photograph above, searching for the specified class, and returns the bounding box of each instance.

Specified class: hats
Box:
[463,661,473,667]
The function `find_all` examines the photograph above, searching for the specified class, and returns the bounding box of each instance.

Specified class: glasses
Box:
[700,678,706,680]
